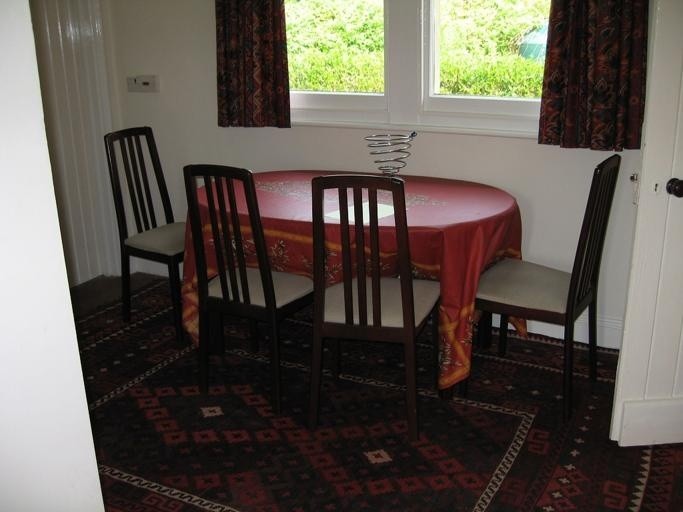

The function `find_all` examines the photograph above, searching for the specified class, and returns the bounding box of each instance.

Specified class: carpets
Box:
[76,277,682,512]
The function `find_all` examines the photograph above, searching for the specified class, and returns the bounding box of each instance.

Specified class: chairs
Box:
[100,125,199,346]
[307,174,444,439]
[440,153,625,426]
[181,161,320,415]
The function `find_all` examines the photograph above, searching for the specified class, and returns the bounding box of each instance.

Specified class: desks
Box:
[177,169,522,441]
